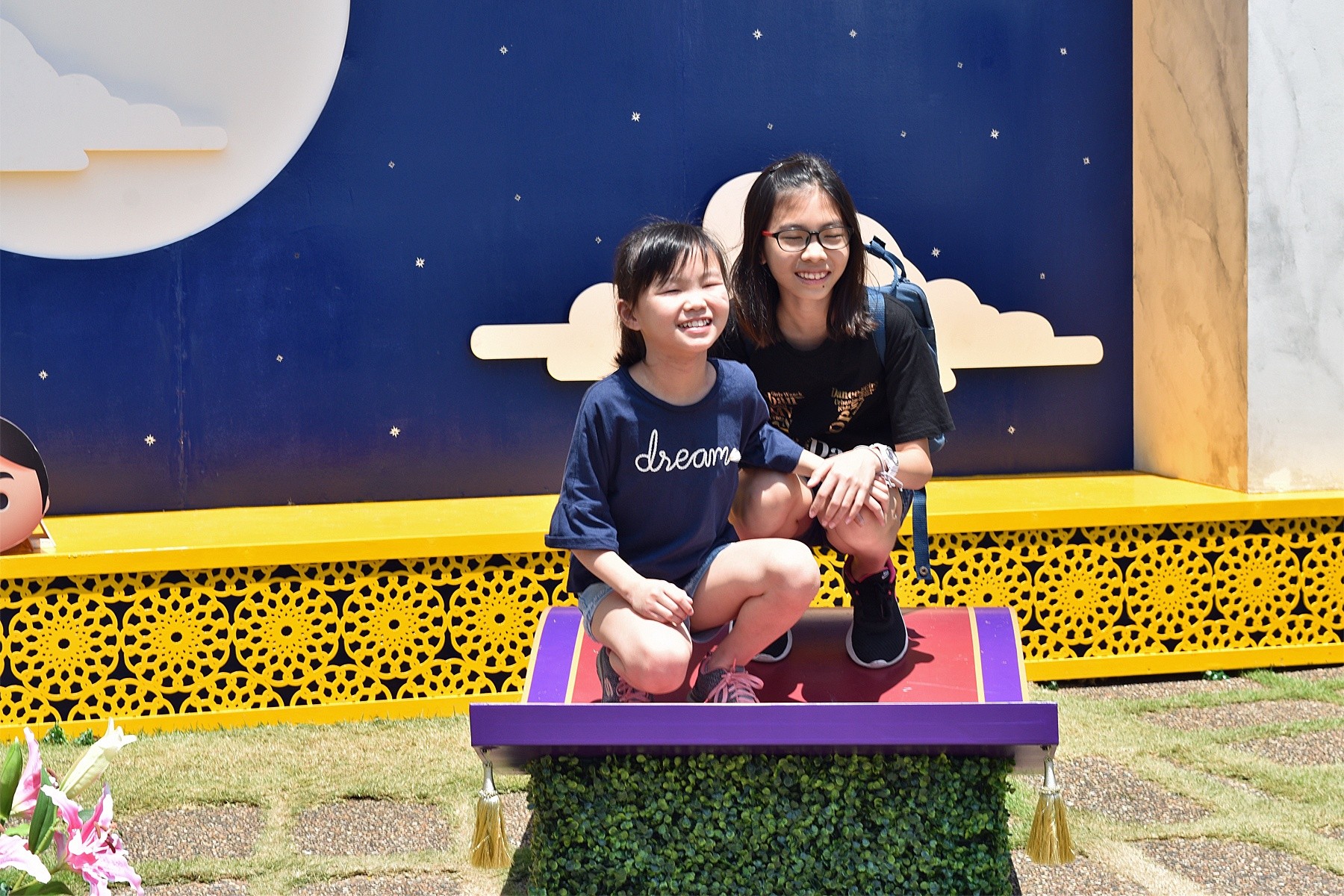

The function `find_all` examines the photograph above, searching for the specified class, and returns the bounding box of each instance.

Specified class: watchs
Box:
[870,443,903,490]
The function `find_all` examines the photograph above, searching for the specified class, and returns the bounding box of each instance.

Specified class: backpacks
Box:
[864,237,945,452]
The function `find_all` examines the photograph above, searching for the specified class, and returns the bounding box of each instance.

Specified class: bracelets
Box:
[853,445,887,482]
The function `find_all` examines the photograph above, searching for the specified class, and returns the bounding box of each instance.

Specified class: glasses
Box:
[763,226,851,253]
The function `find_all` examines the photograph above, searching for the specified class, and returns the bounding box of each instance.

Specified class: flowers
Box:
[0,718,145,896]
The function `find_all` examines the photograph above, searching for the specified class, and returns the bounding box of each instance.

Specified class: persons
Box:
[707,152,954,668]
[544,213,890,703]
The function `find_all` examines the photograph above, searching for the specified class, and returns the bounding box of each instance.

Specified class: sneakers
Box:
[595,645,654,702]
[729,618,793,662]
[687,653,764,703]
[842,554,908,668]
